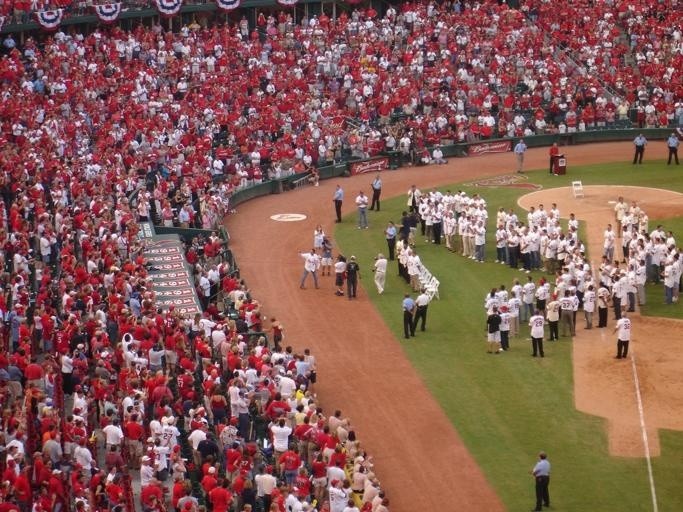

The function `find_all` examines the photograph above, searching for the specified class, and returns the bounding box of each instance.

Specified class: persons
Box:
[0,224,389,512]
[0,0,683,232]
[385,185,682,359]
[532,452,550,512]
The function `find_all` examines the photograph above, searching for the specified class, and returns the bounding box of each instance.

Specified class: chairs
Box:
[572,180,583,197]
[418,264,441,300]
